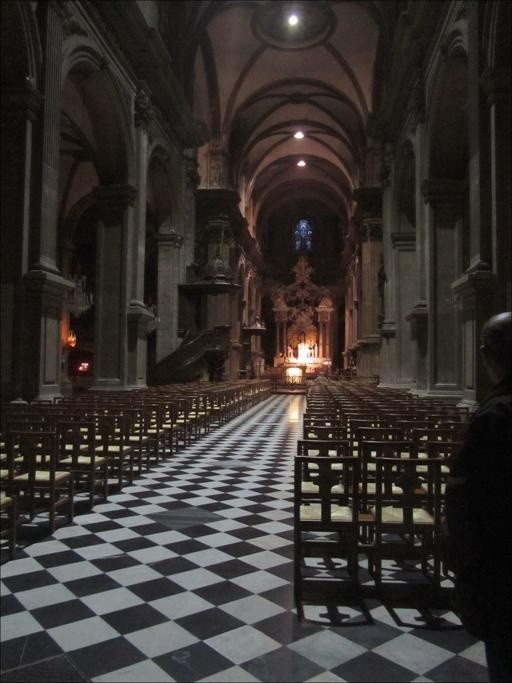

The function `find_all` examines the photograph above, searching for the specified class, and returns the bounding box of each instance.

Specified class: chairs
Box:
[0,378,272,559]
[294,384,483,596]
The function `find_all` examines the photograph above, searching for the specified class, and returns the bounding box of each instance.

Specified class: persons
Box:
[442,312,512,683]
